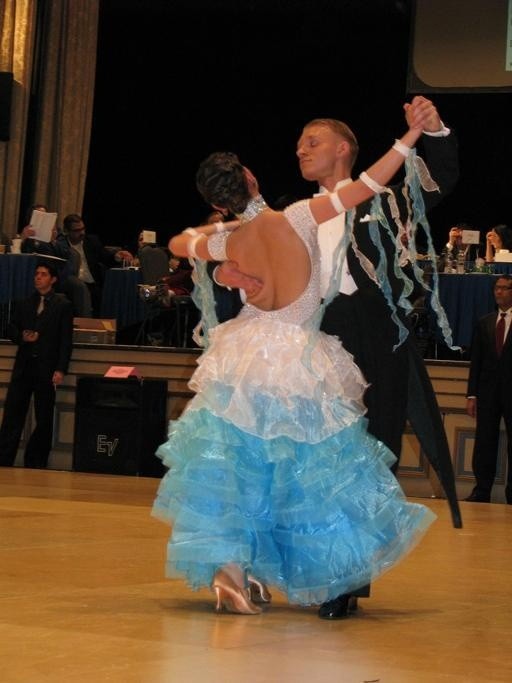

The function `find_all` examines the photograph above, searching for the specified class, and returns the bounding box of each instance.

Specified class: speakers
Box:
[71,373,168,478]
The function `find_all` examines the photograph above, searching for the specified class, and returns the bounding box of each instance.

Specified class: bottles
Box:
[438,249,465,274]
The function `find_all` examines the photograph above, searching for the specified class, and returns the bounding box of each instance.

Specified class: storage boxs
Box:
[72,318,117,343]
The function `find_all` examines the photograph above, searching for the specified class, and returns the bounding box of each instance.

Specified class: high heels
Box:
[246,575,273,604]
[210,569,264,615]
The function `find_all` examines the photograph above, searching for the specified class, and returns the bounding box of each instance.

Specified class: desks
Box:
[1,253,69,344]
[100,267,176,347]
[414,259,511,360]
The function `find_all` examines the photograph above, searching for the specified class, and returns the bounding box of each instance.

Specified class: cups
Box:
[0,244,6,254]
[122,254,133,270]
[10,239,22,254]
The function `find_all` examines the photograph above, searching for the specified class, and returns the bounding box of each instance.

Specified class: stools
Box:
[136,295,218,347]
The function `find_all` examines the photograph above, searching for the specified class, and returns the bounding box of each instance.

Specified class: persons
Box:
[401,223,428,256]
[444,223,486,266]
[486,225,512,262]
[151,103,438,614]
[209,96,459,620]
[465,273,512,505]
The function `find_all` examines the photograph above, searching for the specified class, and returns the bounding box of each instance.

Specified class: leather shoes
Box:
[318,588,360,619]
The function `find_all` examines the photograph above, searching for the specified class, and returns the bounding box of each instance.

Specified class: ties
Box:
[496,312,506,356]
[37,296,44,314]
